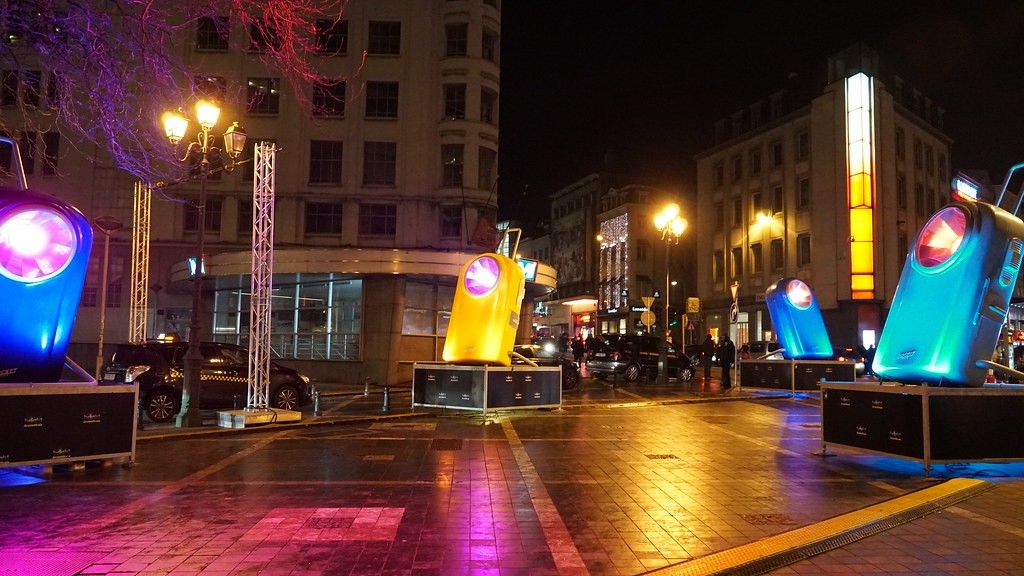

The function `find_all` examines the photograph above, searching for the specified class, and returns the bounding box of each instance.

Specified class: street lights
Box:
[652,203,689,384]
[159,96,247,428]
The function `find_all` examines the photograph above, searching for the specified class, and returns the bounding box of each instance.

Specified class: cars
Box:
[738,339,783,366]
[829,347,865,378]
[531,323,579,360]
[491,344,580,389]
[685,343,735,370]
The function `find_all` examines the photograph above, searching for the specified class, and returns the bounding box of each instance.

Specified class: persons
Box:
[716,343,721,367]
[721,336,735,389]
[985,369,996,384]
[556,331,596,367]
[701,334,714,380]
[530,331,546,337]
[741,345,753,360]
[854,342,877,376]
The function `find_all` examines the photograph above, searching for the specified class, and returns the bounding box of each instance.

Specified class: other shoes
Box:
[721,384,730,388]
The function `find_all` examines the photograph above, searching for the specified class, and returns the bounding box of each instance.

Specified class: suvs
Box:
[101,340,318,424]
[585,332,697,383]
